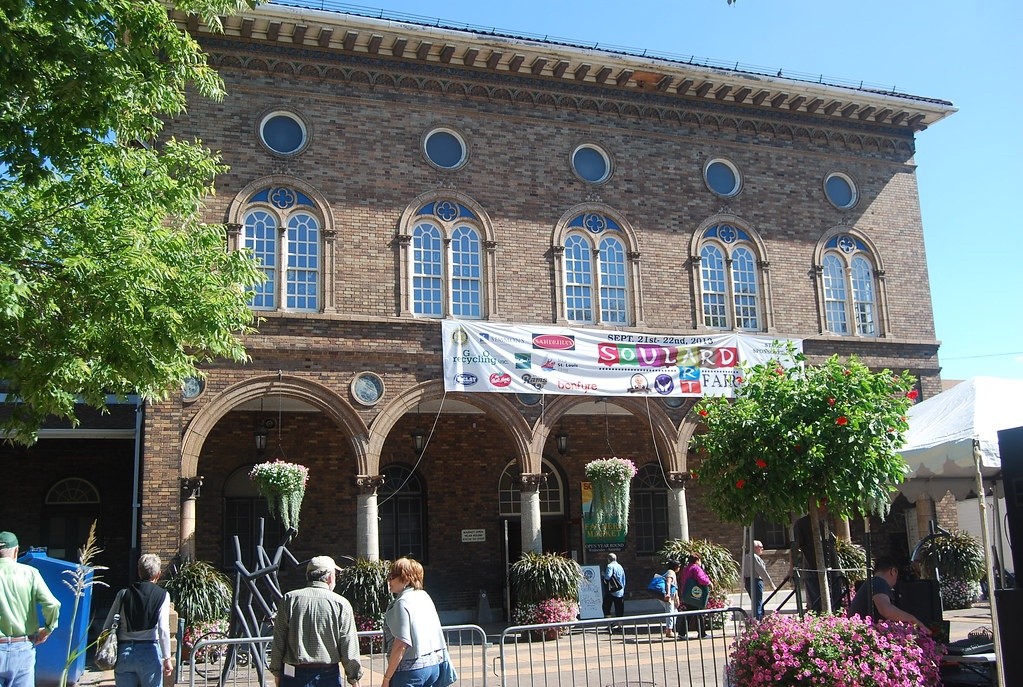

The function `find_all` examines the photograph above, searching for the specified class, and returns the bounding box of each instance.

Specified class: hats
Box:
[608,553,617,561]
[306,556,344,574]
[0,531,19,549]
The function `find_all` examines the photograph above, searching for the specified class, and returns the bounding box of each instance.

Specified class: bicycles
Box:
[190,605,274,681]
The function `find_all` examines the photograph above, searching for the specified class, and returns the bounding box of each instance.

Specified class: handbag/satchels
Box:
[432,649,457,687]
[94,613,121,670]
[647,571,677,604]
[682,578,710,609]
[606,575,623,593]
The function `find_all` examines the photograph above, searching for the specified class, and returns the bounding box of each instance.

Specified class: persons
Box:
[269,555,365,687]
[379,557,444,687]
[678,555,714,640]
[790,488,844,615]
[0,532,61,687]
[744,540,777,624]
[601,553,626,628]
[101,553,173,687]
[846,555,934,636]
[647,561,679,639]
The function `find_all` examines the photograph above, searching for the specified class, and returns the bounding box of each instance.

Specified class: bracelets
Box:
[383,675,391,681]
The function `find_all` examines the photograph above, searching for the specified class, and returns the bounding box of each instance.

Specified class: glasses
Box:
[387,573,401,580]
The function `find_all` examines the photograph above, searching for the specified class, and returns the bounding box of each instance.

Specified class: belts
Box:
[296,664,333,668]
[0,636,29,644]
[119,640,158,645]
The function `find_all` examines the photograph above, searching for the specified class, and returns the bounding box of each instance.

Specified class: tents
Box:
[849,382,1023,687]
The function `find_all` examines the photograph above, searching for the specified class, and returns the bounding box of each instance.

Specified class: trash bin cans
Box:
[18,548,94,687]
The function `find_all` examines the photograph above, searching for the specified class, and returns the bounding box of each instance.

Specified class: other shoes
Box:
[666,633,676,638]
[678,635,689,640]
[604,614,611,618]
[699,634,713,639]
